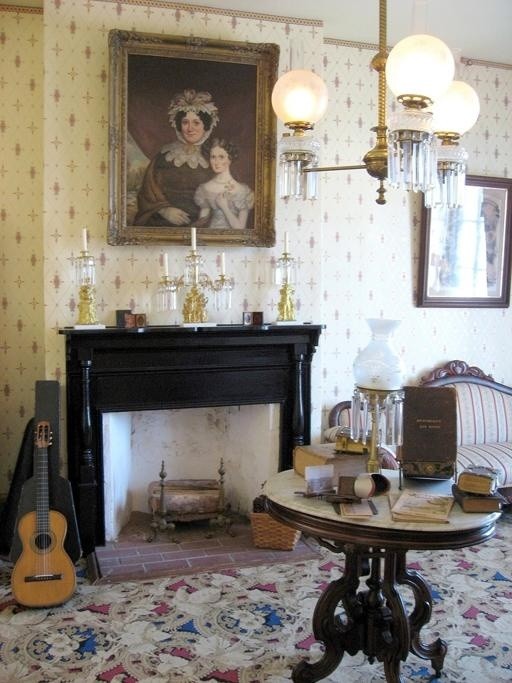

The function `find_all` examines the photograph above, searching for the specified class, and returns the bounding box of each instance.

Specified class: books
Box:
[392,488,456,518]
[339,504,372,518]
[341,501,378,516]
[452,482,509,513]
[293,442,378,489]
[388,493,448,522]
[401,387,461,477]
[458,465,502,495]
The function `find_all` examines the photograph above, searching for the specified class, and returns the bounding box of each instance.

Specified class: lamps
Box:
[433,82,480,138]
[354,318,404,474]
[271,68,329,126]
[386,36,448,108]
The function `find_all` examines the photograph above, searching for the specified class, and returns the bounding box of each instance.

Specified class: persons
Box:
[193,140,253,231]
[136,90,255,226]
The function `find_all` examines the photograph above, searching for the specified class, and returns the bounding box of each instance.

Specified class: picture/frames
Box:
[418,175,511,307]
[108,30,280,249]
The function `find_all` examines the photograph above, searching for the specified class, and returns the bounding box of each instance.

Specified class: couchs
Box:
[328,363,512,512]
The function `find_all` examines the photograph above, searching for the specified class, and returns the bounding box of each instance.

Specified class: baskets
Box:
[250,510,302,551]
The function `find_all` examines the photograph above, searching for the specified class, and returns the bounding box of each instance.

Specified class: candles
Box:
[160,257,169,282]
[191,227,196,254]
[81,230,90,256]
[282,230,290,256]
[219,251,226,278]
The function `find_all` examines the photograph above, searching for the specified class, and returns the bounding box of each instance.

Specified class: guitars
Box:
[10,422,75,606]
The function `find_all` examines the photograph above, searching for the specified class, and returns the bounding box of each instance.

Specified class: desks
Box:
[264,465,499,683]
